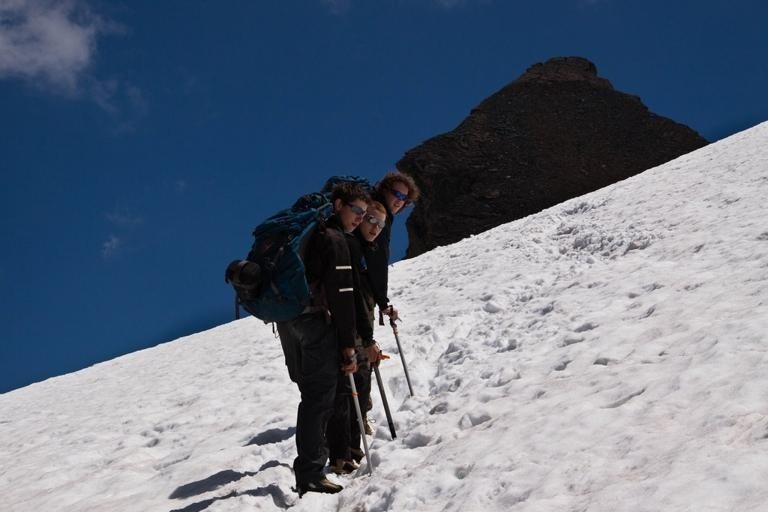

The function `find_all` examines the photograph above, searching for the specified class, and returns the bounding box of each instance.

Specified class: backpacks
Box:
[291,175,375,212]
[225,203,335,324]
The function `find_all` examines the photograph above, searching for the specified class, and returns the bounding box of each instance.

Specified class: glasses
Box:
[365,214,386,228]
[387,187,408,201]
[342,201,368,217]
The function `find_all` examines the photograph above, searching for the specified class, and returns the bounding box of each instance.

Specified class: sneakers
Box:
[300,474,343,493]
[330,417,372,473]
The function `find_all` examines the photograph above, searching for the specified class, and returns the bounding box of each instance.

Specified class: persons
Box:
[276,181,372,498]
[363,170,423,435]
[326,200,392,474]
